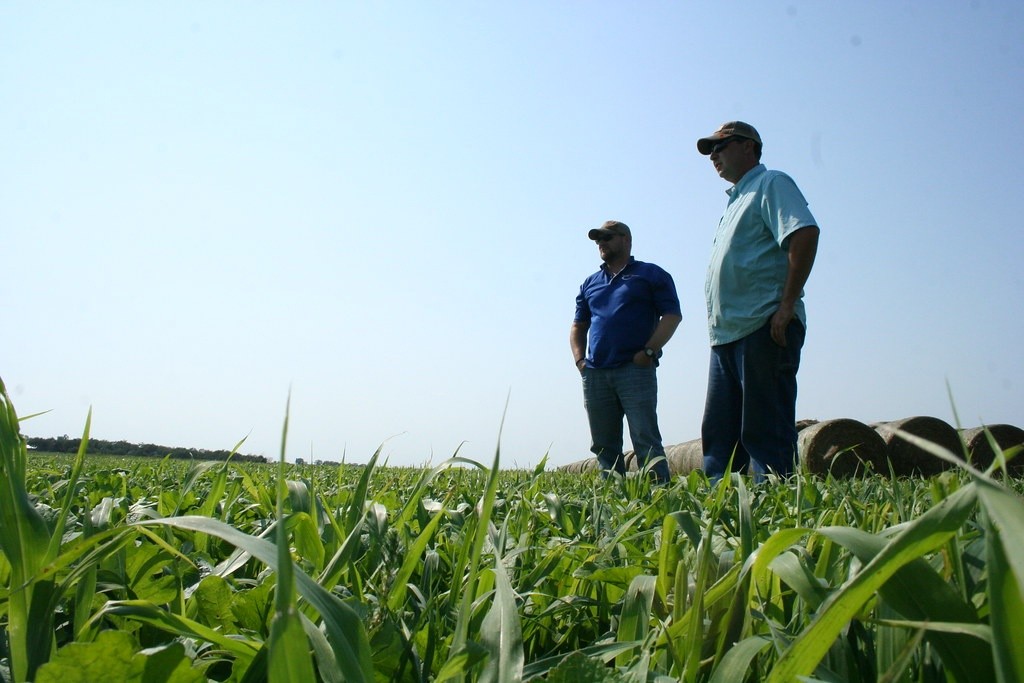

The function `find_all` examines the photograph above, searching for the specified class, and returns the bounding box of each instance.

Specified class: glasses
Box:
[595,234,616,245]
[710,136,737,154]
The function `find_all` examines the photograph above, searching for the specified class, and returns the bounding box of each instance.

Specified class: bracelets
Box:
[575,357,584,367]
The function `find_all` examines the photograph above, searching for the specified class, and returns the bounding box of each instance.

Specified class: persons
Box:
[697,119,820,489]
[569,220,686,491]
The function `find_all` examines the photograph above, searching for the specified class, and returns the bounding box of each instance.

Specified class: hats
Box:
[696,120,763,155]
[588,220,632,240]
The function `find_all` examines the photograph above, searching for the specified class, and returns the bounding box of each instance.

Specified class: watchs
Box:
[642,347,658,359]
